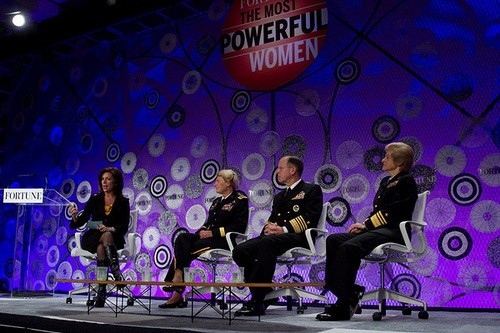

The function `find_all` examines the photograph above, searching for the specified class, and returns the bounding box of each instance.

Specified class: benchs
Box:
[54,278,325,324]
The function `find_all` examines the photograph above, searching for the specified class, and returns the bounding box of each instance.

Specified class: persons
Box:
[159,169,249,308]
[231,156,323,317]
[317,143,419,321]
[69,168,131,307]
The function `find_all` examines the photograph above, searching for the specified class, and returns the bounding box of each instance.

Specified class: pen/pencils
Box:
[96,223,100,227]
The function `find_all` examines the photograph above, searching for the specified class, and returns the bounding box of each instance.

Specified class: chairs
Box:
[66,209,139,305]
[264,202,331,313]
[182,207,255,311]
[355,190,431,321]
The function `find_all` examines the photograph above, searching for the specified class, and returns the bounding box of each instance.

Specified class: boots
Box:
[104,245,127,290]
[88,258,110,308]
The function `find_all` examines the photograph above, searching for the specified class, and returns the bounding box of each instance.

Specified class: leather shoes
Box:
[316,286,365,321]
[265,285,275,295]
[234,304,266,316]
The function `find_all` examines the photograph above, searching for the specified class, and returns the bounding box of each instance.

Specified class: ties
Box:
[218,199,225,206]
[284,187,291,199]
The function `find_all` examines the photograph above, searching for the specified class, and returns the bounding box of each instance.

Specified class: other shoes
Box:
[158,298,187,309]
[162,281,185,292]
